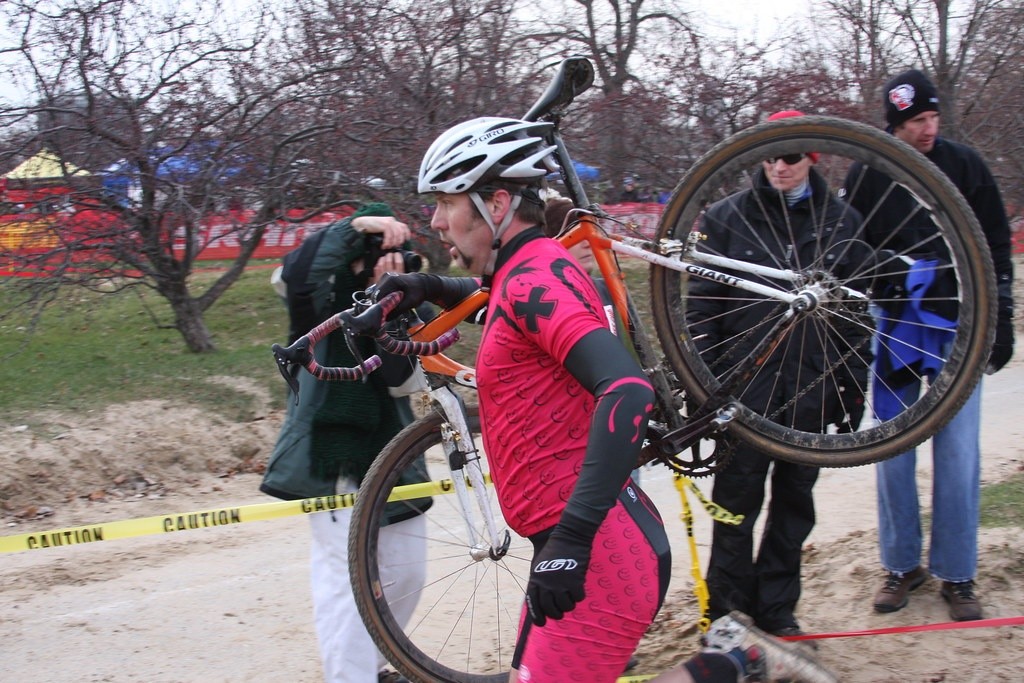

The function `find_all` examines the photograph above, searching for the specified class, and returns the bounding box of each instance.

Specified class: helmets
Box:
[418,117,560,194]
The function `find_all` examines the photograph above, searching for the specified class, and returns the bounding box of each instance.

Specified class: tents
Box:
[0,139,273,210]
[546,156,601,204]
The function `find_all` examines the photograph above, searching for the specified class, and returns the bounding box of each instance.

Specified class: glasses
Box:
[767,152,809,166]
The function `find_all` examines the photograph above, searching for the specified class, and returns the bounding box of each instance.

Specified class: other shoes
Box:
[378,669,410,683]
[700,610,839,683]
[768,627,816,650]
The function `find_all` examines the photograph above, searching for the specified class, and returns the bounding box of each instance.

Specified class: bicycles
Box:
[274,58,1000,683]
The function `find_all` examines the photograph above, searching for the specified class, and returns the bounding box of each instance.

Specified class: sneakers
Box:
[941,579,981,624]
[874,566,927,612]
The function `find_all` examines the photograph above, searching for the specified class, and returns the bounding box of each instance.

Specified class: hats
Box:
[767,112,819,163]
[883,71,941,125]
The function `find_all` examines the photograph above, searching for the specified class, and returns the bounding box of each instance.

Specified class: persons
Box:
[372,114,840,683]
[837,68,1017,622]
[259,201,436,683]
[620,178,672,204]
[684,110,874,647]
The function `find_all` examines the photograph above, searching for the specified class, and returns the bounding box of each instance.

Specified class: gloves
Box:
[833,368,867,435]
[526,510,598,626]
[985,283,1015,375]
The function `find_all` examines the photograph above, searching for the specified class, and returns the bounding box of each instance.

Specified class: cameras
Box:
[364,231,422,274]
